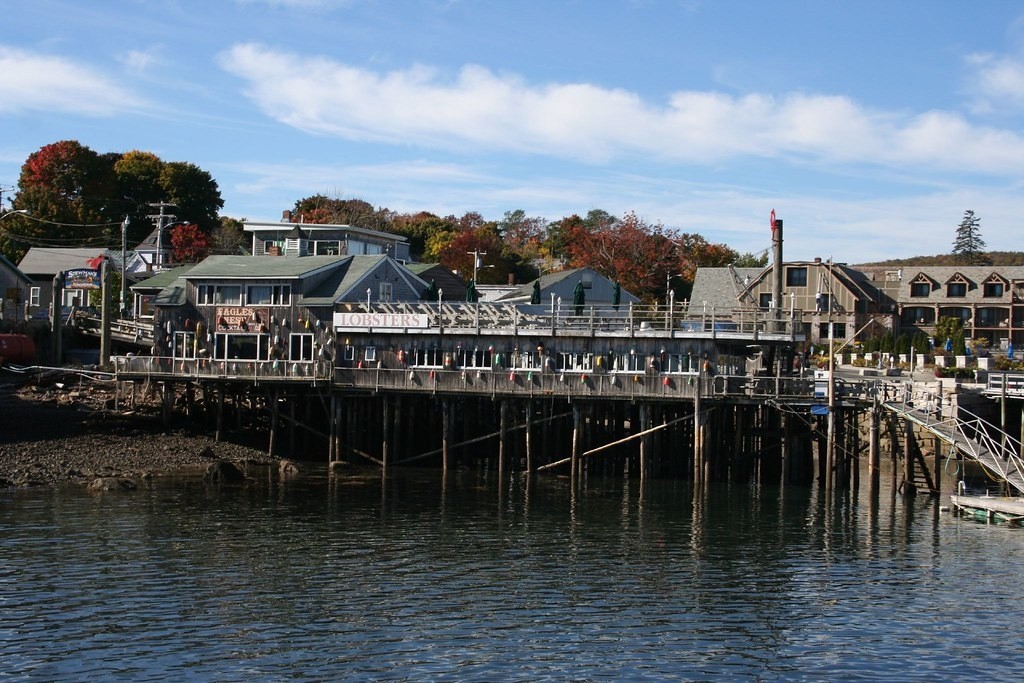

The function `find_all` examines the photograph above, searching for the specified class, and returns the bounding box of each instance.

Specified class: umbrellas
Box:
[530,280,541,305]
[945,336,952,354]
[611,281,621,330]
[1008,342,1014,358]
[572,280,585,330]
[466,278,475,303]
[429,279,438,301]
[966,338,982,356]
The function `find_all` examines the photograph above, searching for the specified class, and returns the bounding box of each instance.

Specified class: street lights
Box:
[155,220,189,271]
[670,290,675,339]
[666,266,682,327]
[557,296,562,329]
[438,288,443,335]
[366,287,373,314]
[791,292,796,340]
[550,293,556,335]
[472,264,495,291]
